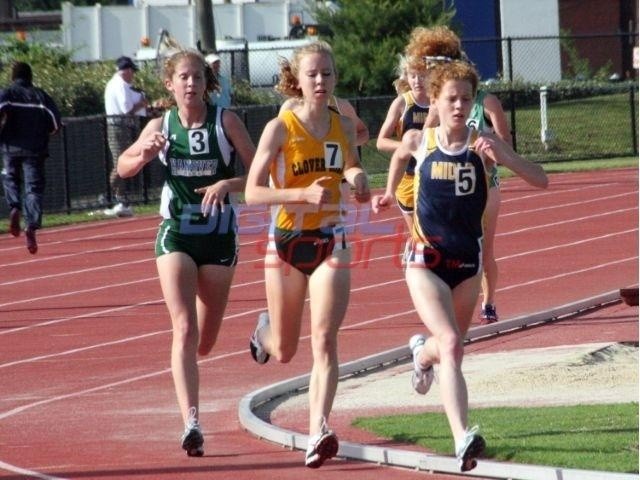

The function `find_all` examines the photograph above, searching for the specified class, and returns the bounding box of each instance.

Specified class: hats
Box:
[204,53,221,65]
[113,54,140,73]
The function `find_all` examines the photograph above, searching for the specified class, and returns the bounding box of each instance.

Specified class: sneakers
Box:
[250,311,276,368]
[477,301,499,321]
[24,226,39,254]
[8,207,22,239]
[454,428,488,475]
[96,192,132,217]
[404,334,436,396]
[177,423,207,459]
[300,430,339,469]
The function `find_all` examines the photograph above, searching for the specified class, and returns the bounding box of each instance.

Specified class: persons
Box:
[421,84,513,324]
[116,51,255,457]
[377,52,435,268]
[249,42,369,470]
[0,62,62,254]
[369,23,549,472]
[204,52,237,112]
[273,89,369,151]
[103,56,149,218]
